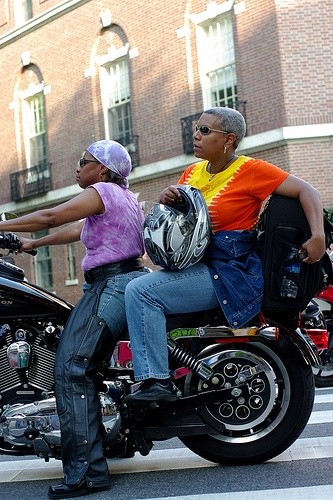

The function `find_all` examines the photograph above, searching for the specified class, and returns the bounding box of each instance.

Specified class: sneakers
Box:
[125,378,181,402]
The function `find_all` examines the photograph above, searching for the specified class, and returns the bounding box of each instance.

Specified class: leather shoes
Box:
[48,480,111,500]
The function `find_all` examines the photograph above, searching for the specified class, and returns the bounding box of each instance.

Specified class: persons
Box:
[0,142,156,499]
[123,107,327,404]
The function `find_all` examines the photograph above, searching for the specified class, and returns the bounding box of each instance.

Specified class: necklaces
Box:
[208,156,235,182]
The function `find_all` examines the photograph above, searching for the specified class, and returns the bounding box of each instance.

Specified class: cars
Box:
[299,251,333,388]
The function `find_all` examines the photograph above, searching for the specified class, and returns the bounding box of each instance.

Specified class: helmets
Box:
[143,186,210,271]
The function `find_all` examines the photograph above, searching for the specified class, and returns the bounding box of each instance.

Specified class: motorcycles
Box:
[0,211,322,466]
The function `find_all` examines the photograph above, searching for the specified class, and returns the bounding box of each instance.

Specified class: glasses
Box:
[194,125,230,135]
[80,159,99,168]
